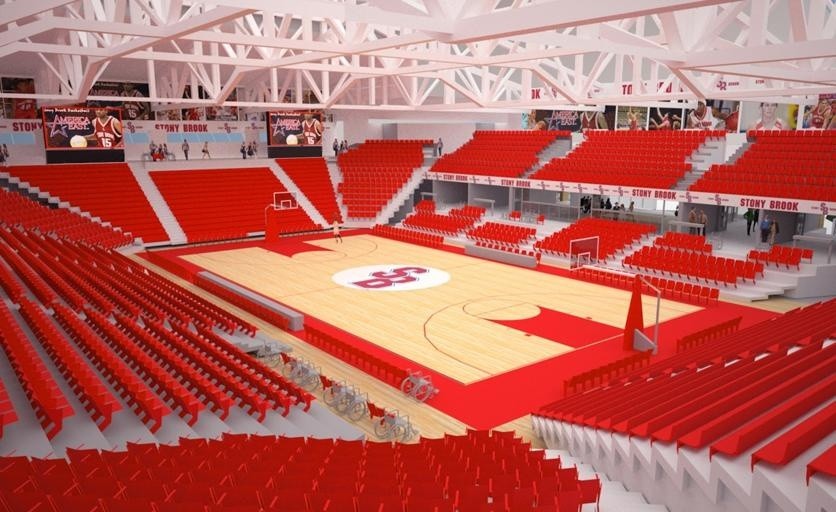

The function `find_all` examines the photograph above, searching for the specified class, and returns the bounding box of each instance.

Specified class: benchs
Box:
[1,434,603,512]
[1,156,345,247]
[404,128,833,294]
[2,243,317,442]
[337,137,434,225]
[528,296,836,512]
